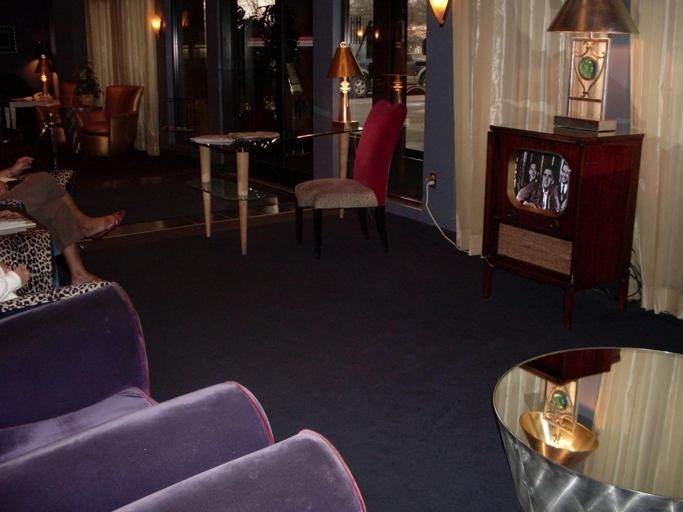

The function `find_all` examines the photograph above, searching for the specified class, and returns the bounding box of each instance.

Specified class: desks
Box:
[490,346,682,512]
[190,129,281,256]
[1,93,56,131]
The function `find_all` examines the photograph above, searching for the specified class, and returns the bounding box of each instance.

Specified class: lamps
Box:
[546,1,644,135]
[324,38,364,130]
[513,349,622,464]
[31,52,55,102]
[426,0,455,28]
[147,9,164,43]
[380,42,418,127]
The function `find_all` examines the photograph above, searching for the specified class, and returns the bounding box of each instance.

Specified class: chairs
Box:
[1,279,274,512]
[292,97,408,261]
[112,425,372,512]
[2,168,117,316]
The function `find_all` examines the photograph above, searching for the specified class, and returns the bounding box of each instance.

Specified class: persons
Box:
[0,155,127,285]
[514,159,570,210]
[0,255,33,304]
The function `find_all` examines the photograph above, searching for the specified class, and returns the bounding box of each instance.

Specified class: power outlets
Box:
[428,171,439,189]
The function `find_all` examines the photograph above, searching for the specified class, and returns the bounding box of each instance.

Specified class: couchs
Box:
[38,80,146,159]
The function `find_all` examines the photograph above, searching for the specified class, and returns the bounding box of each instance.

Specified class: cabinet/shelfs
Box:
[472,122,646,334]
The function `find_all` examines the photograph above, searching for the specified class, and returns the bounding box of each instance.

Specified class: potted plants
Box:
[75,66,99,109]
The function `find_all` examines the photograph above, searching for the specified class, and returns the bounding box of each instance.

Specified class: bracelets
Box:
[7,167,17,177]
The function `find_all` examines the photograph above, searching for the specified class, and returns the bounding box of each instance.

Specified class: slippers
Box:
[92,206,128,242]
